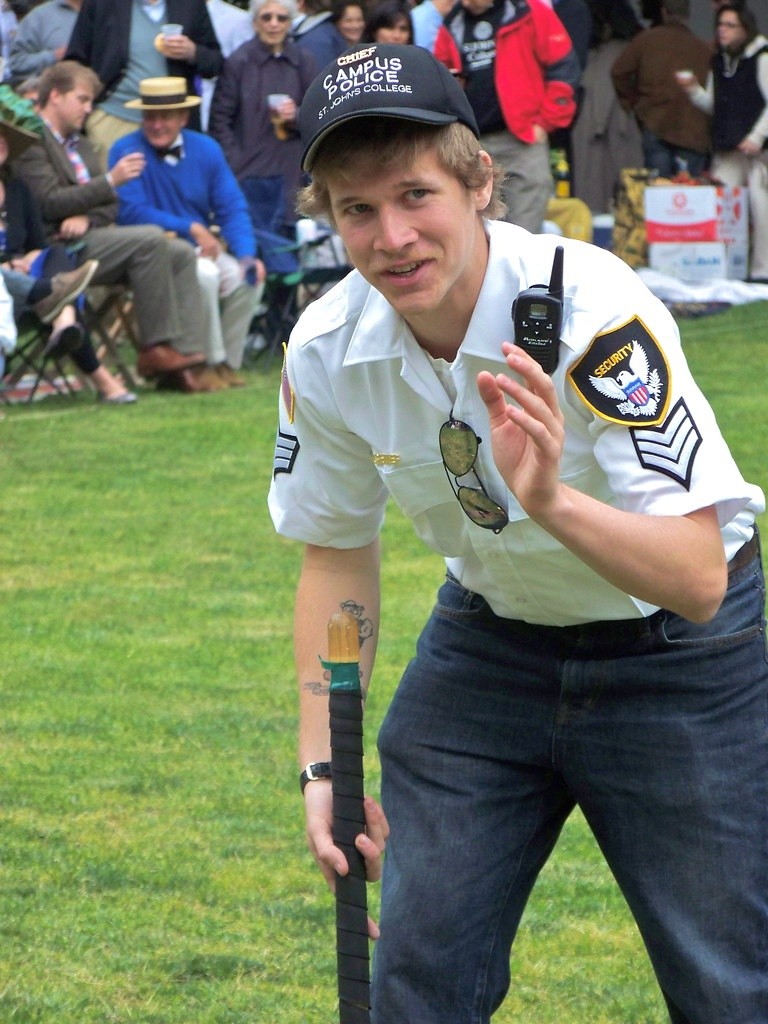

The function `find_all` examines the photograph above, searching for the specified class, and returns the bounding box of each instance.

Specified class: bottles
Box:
[556,155,570,199]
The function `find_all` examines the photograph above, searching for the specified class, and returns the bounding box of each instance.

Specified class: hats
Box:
[300,41,479,178]
[122,77,204,110]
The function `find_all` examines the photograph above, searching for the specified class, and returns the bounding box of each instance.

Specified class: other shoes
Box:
[137,347,205,377]
[44,326,81,361]
[97,387,138,406]
[157,368,208,392]
[199,364,246,390]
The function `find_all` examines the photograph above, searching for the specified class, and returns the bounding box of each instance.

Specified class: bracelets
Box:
[299,761,332,794]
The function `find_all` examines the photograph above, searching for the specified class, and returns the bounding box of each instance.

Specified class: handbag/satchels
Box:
[613,167,674,272]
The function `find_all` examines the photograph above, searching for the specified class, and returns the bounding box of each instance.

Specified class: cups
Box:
[677,70,693,86]
[160,22,182,37]
[268,94,289,123]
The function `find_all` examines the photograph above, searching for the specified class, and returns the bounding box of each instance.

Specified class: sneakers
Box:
[35,259,101,323]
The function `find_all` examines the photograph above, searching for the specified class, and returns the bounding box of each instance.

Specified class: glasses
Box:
[440,420,510,538]
[260,12,290,25]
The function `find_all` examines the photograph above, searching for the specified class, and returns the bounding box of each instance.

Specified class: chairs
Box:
[1,231,352,405]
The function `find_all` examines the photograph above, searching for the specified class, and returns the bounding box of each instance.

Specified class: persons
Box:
[266,45,767,1024]
[0,1,768,410]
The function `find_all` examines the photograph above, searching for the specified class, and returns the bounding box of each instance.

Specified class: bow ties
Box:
[157,145,181,160]
